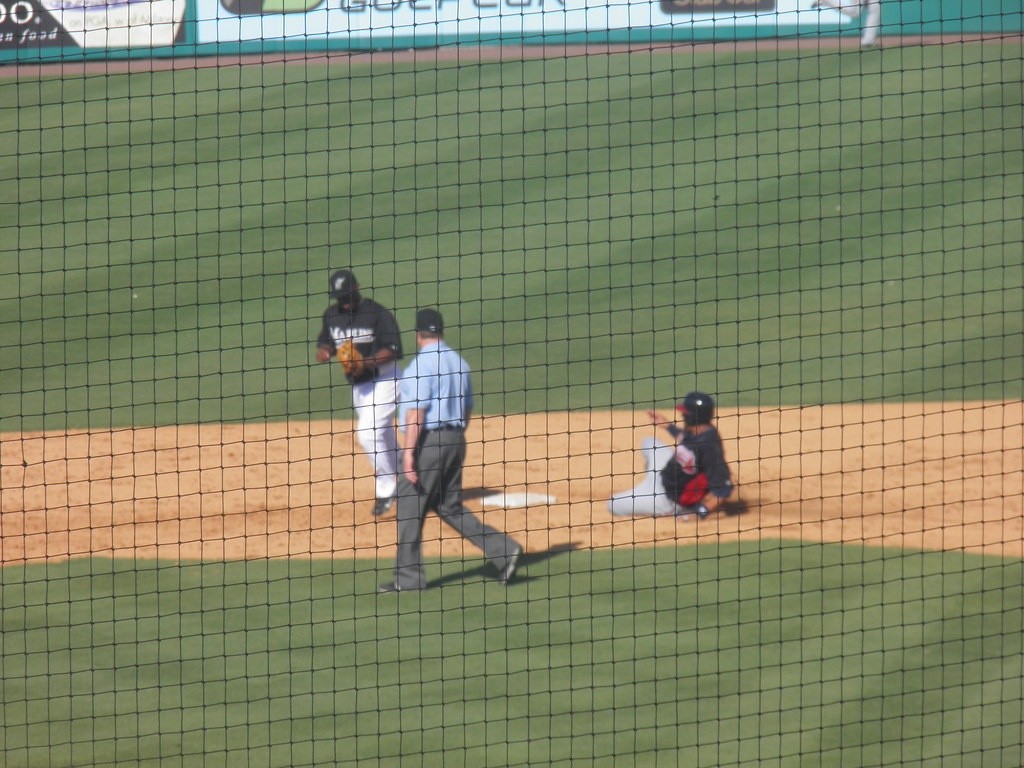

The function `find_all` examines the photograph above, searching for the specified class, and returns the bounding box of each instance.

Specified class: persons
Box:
[608,392,733,522]
[316,269,405,514]
[380,309,523,592]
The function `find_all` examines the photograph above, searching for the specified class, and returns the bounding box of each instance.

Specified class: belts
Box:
[423,426,458,432]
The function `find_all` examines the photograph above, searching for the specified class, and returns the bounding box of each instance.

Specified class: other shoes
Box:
[379,582,396,593]
[500,544,522,586]
[372,497,393,515]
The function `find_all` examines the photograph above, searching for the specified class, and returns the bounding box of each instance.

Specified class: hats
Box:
[329,268,358,298]
[416,308,444,333]
[675,392,715,417]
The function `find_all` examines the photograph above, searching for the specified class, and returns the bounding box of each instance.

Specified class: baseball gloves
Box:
[337,340,365,378]
